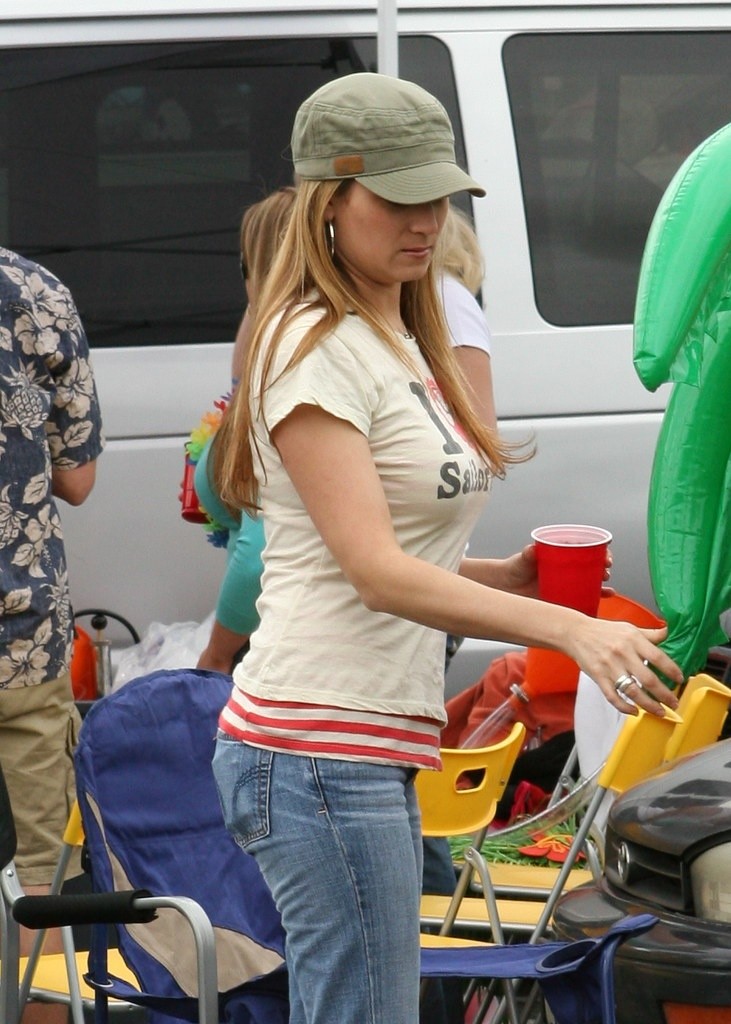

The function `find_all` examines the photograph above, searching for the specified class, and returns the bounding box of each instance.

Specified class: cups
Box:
[531,522,612,620]
[180,441,213,524]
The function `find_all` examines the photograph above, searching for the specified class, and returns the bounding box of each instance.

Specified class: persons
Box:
[193,72,684,1024]
[0,244,107,1004]
[612,799,731,825]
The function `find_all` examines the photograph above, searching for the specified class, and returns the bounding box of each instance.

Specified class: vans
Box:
[1,5,731,715]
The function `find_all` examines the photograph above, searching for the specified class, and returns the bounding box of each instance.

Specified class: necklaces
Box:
[394,325,410,340]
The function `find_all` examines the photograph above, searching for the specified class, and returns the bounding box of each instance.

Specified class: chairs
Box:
[0,673,731,1024]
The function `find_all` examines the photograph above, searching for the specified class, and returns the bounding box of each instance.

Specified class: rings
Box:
[620,678,636,693]
[615,674,631,688]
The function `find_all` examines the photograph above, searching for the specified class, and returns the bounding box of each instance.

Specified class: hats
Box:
[291,72,486,205]
